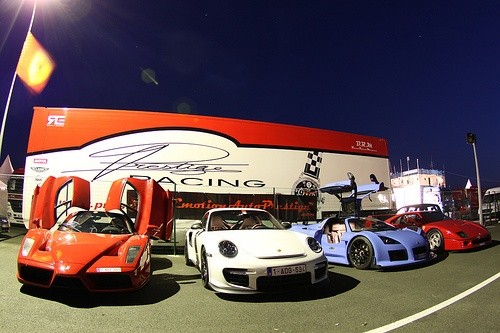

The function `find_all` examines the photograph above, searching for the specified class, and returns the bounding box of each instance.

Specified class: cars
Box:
[284,172,433,269]
[366,204,491,253]
[184,208,328,297]
[0,214,9,231]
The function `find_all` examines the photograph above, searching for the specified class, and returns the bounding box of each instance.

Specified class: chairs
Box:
[75,214,96,231]
[239,216,256,229]
[210,214,228,229]
[110,219,126,226]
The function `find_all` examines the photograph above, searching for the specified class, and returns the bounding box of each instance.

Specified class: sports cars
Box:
[17,175,174,295]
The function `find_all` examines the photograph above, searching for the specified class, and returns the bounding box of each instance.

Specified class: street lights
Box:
[465,132,484,227]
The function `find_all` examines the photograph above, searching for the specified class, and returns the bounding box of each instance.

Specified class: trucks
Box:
[5,169,24,225]
[478,187,500,221]
